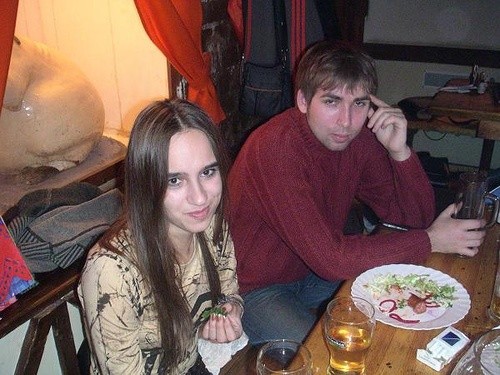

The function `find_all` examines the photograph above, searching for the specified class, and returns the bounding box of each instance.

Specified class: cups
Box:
[256,338,312,375]
[451,330,500,375]
[453,171,500,258]
[324,297,376,375]
[488,259,500,327]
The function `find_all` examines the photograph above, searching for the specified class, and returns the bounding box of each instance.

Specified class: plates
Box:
[350,264,471,330]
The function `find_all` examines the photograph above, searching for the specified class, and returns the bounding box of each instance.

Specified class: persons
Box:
[77,98,250,375]
[222,40,487,367]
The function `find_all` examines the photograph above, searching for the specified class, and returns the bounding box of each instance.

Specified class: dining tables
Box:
[283,202,500,374]
[425,79,500,182]
